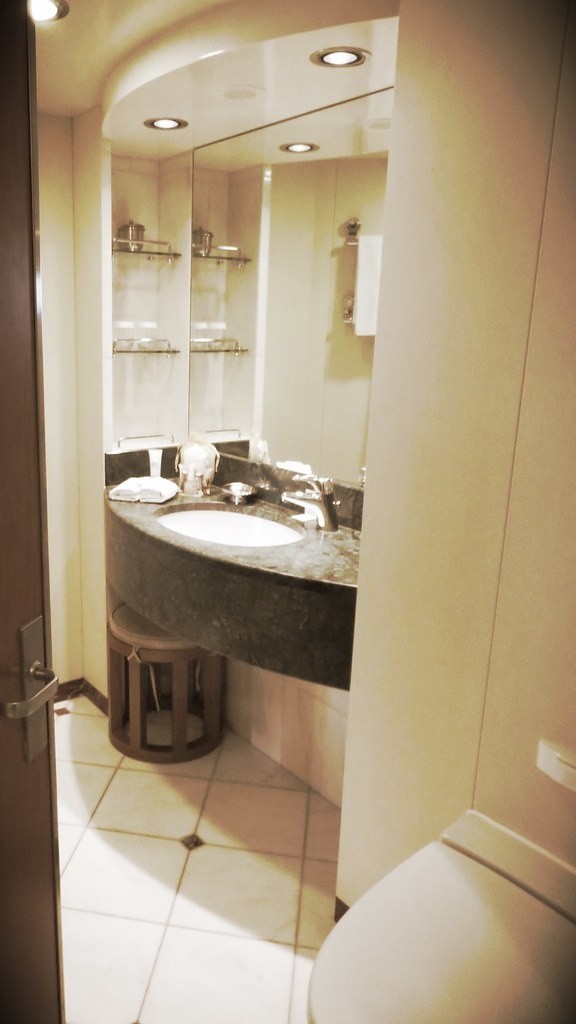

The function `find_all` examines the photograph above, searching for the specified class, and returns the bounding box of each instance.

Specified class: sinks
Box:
[153,503,308,547]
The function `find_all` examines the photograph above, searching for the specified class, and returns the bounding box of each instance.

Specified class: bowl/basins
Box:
[222,483,257,503]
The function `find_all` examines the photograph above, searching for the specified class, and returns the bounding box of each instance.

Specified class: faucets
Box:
[281,474,339,532]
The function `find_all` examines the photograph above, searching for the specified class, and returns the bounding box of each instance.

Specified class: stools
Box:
[104,601,223,765]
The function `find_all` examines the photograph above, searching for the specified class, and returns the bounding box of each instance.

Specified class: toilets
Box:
[305,809,576,1024]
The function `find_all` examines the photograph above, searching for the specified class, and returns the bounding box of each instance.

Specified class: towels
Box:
[108,477,179,503]
[353,235,383,337]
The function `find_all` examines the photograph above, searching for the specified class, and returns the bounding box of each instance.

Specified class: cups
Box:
[118,219,145,251]
[192,227,213,257]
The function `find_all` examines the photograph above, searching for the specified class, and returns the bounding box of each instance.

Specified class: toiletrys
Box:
[148,448,163,478]
[179,460,203,497]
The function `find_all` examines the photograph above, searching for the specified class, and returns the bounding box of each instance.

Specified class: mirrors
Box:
[189,87,394,493]
